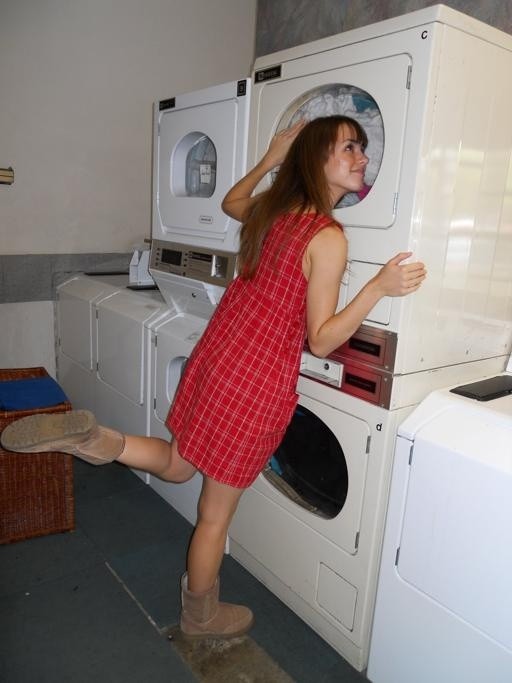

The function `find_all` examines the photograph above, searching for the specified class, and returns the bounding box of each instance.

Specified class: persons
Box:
[0,115,427,638]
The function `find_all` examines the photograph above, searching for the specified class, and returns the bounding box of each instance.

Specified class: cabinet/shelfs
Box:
[365,372,511,682]
[1,366,75,545]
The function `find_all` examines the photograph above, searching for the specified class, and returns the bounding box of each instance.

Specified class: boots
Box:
[1,408,125,466]
[179,571,255,641]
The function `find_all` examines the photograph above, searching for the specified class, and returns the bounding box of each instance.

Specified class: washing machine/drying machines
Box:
[149,311,229,555]
[151,78,249,254]
[228,374,511,672]
[247,2,512,373]
